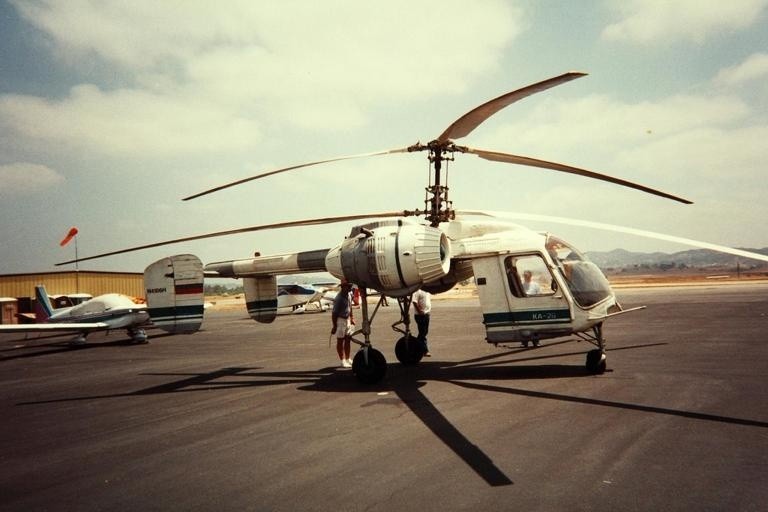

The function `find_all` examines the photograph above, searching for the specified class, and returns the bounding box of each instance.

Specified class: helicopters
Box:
[56,72,768,382]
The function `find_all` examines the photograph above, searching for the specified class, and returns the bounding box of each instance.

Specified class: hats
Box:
[338,278,353,287]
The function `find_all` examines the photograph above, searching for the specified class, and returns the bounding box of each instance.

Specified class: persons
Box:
[381,292,389,306]
[330,276,357,368]
[412,285,433,356]
[549,249,565,292]
[522,271,542,348]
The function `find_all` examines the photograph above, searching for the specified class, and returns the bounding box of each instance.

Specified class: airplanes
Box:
[216,281,380,316]
[0,285,215,345]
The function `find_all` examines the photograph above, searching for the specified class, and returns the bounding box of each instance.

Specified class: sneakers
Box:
[340,359,357,367]
